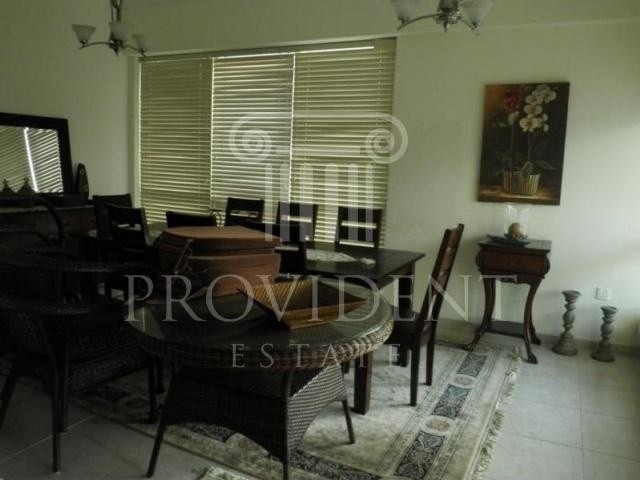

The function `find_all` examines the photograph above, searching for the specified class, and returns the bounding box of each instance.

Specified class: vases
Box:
[502,170,523,194]
[517,172,540,195]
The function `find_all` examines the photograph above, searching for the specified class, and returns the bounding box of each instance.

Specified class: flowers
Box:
[493,91,523,170]
[519,83,559,178]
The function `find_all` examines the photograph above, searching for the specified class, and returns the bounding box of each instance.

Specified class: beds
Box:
[124,277,392,480]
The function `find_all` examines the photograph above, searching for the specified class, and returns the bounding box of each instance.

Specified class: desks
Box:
[472,238,553,365]
[275,238,426,415]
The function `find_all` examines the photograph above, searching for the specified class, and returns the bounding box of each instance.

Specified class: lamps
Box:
[391,0,492,35]
[70,0,151,62]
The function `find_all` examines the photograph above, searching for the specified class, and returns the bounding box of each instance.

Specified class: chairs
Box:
[378,222,462,408]
[144,362,356,480]
[0,246,155,474]
[0,196,70,260]
[91,191,385,255]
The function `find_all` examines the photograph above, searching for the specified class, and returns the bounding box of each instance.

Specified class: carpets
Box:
[72,329,524,480]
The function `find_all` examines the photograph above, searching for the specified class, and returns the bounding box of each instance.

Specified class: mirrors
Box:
[0,112,76,197]
